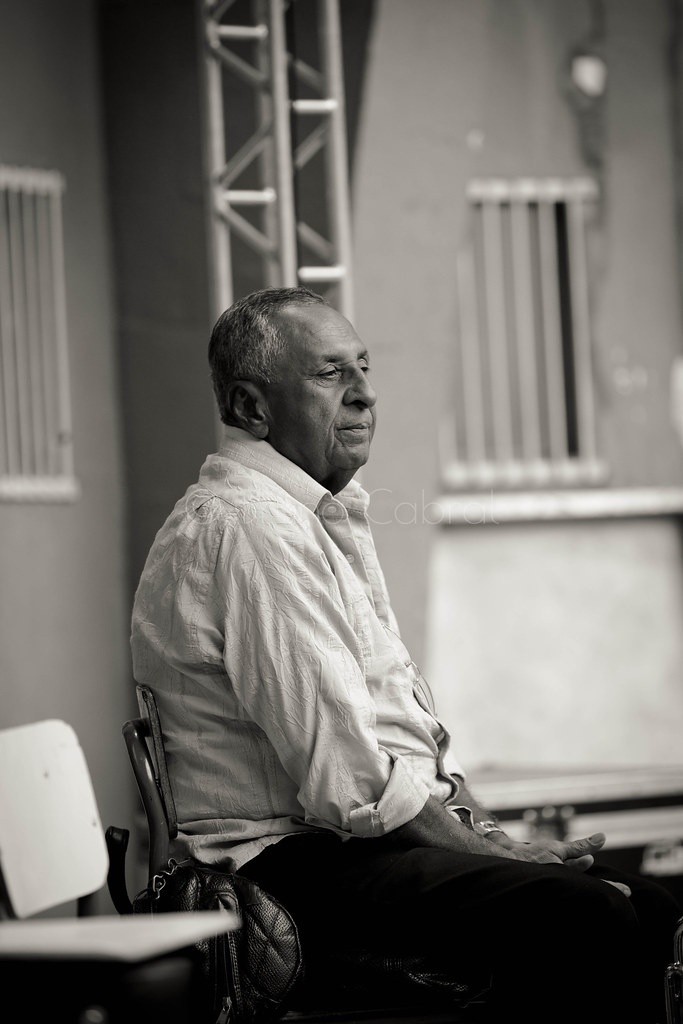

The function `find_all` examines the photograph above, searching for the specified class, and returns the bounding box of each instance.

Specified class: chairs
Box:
[0,716,132,922]
[122,680,181,887]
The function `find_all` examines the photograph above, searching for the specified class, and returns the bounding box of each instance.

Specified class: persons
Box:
[129,287,683,1024]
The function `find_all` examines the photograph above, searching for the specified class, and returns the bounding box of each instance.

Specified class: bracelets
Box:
[475,821,508,837]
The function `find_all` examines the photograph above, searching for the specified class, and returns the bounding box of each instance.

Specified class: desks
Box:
[0,911,242,966]
